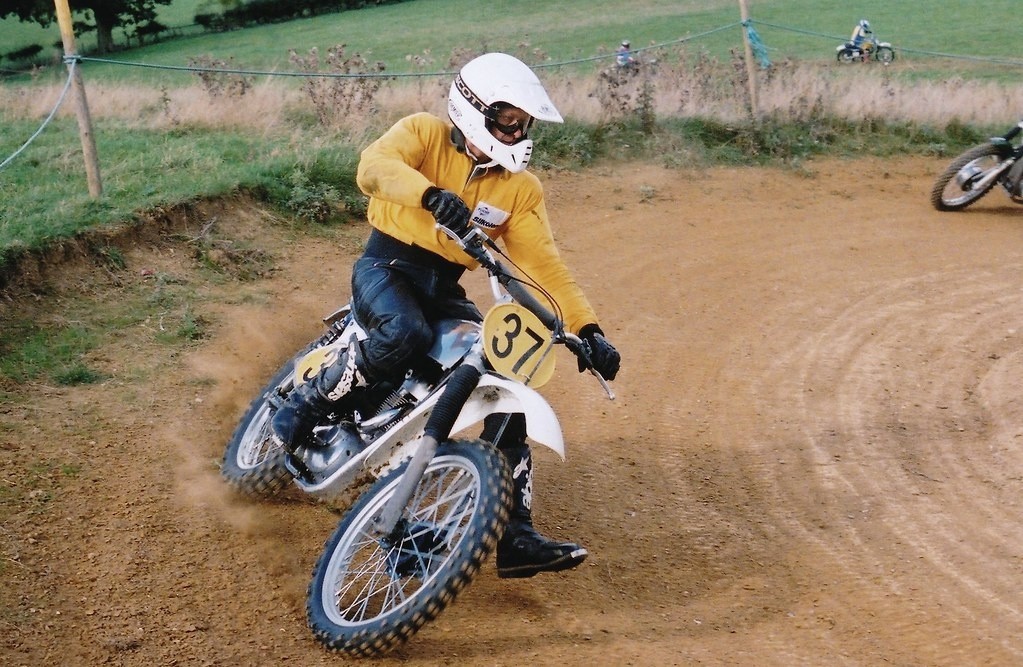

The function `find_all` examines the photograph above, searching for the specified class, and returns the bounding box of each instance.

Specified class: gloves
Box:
[421,185,474,240]
[578,323,620,381]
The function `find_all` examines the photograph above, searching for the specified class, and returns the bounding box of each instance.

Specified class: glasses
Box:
[491,105,535,136]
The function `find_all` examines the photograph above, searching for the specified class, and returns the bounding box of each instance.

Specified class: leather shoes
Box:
[496,443,589,579]
[271,332,395,450]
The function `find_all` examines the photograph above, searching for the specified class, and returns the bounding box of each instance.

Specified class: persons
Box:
[617,40,639,81]
[267,52,621,580]
[849,19,873,66]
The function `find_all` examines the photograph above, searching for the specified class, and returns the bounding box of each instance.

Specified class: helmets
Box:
[447,52,564,174]
[860,20,869,31]
[622,41,630,48]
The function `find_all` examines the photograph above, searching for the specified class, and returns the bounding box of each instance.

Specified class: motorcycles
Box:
[216,194,614,660]
[836,33,894,64]
[930,119,1023,211]
